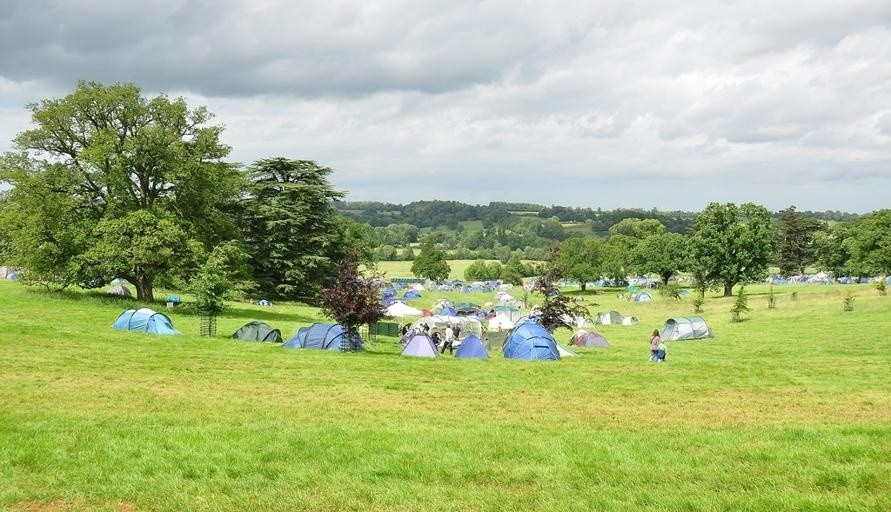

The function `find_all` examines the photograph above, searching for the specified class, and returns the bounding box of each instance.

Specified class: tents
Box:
[420,315,482,342]
[597,279,687,302]
[231,321,283,343]
[107,285,132,297]
[372,279,425,315]
[280,322,363,351]
[110,307,182,335]
[456,336,488,357]
[568,331,608,347]
[660,316,714,340]
[559,310,637,329]
[401,332,440,358]
[502,320,560,360]
[421,293,543,329]
[426,279,512,299]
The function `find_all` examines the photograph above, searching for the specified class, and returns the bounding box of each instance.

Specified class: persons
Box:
[650,329,667,362]
[402,322,460,355]
